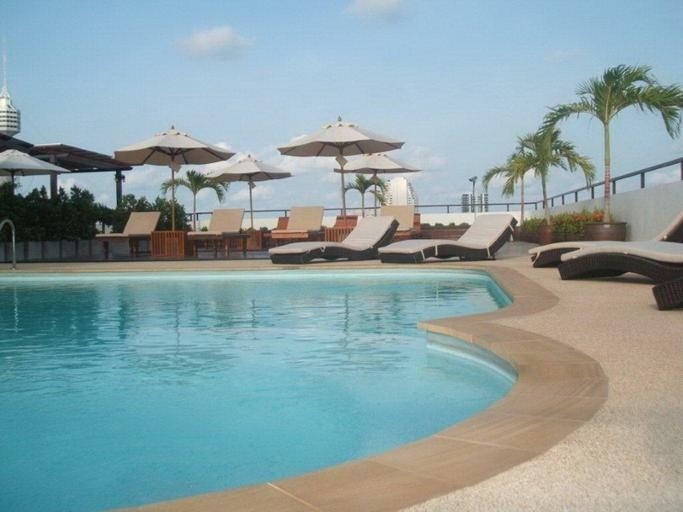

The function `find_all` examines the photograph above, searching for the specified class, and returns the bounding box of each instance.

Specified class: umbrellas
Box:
[332,152,422,217]
[112,129,236,231]
[274,116,405,229]
[0,148,72,196]
[202,155,295,230]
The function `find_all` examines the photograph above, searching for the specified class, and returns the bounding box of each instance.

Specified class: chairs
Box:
[558,240,682,288]
[529,209,683,267]
[260,202,420,261]
[187,208,251,261]
[96,210,161,261]
[377,213,518,264]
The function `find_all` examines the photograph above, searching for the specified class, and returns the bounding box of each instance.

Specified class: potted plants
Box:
[531,68,683,240]
[500,127,597,245]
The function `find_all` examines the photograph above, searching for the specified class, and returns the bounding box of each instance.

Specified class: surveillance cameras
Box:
[468,177,474,181]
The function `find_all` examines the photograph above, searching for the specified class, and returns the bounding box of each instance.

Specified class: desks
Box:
[147,229,194,259]
